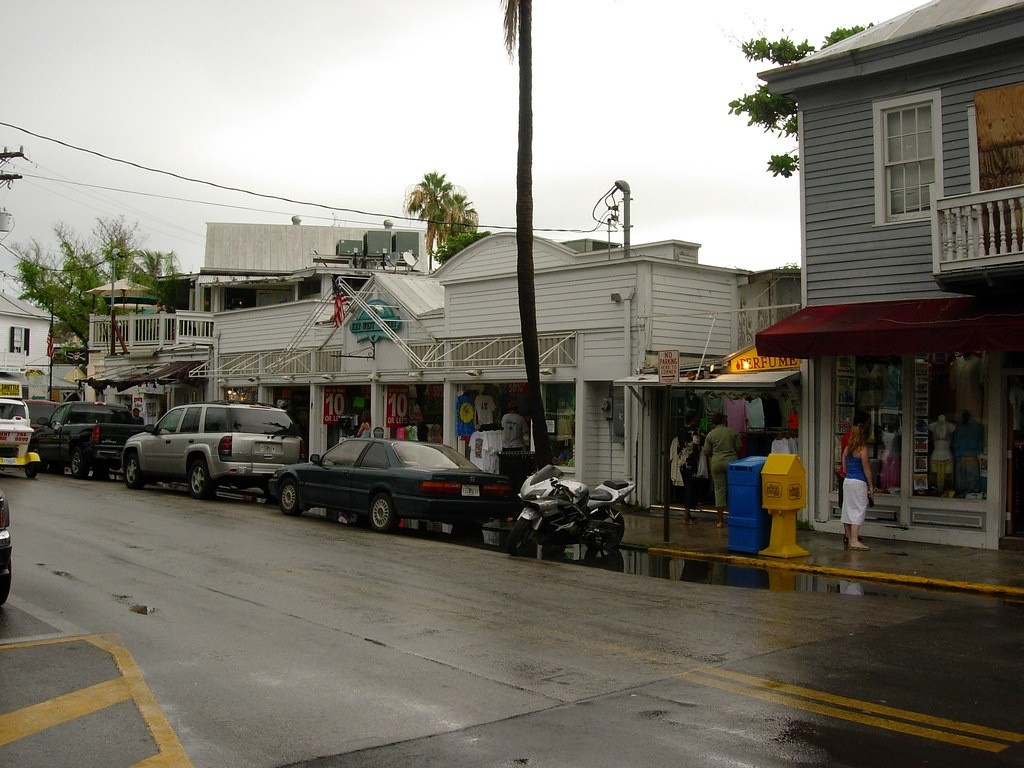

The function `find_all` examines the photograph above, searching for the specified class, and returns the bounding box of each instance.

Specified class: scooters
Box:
[0,398,40,478]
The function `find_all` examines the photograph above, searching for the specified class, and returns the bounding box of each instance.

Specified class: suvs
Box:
[122,401,301,499]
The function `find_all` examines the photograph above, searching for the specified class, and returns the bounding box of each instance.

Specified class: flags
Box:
[332,294,348,327]
[46,323,53,356]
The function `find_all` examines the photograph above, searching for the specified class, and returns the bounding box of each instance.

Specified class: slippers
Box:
[849,544,870,551]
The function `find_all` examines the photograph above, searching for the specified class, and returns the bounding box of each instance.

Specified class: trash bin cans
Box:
[494,451,536,501]
[726,456,768,557]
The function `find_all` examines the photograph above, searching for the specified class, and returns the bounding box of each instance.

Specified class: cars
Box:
[24,400,60,429]
[269,438,513,533]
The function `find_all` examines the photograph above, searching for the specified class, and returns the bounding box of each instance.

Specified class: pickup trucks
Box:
[27,401,145,479]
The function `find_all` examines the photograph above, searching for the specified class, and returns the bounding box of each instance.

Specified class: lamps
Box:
[703,363,714,378]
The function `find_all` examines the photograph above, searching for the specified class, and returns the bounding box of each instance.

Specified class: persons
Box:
[928,414,957,492]
[703,412,743,528]
[154,301,164,336]
[676,410,705,525]
[878,425,901,491]
[501,400,529,451]
[951,410,984,498]
[840,422,875,551]
[838,412,870,543]
[132,408,144,425]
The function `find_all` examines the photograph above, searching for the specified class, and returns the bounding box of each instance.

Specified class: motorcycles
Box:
[506,465,636,555]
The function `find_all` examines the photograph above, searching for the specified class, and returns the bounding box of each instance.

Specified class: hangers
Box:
[775,431,798,440]
[462,389,499,396]
[704,390,791,400]
